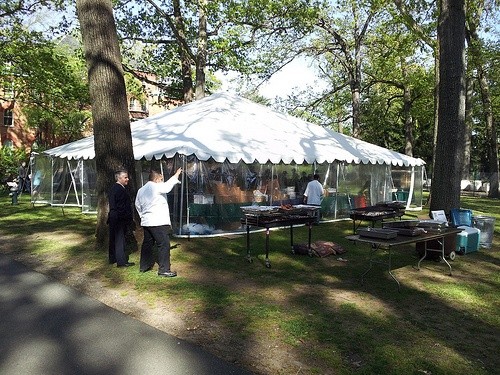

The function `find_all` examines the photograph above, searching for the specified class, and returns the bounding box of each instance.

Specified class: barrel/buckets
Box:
[470,216,495,249]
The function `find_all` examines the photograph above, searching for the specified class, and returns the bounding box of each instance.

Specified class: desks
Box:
[349,211,405,235]
[345,228,464,292]
[320,196,346,212]
[242,216,318,270]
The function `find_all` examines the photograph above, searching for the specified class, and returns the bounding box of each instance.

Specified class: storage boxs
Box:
[188,187,295,229]
[453,206,480,254]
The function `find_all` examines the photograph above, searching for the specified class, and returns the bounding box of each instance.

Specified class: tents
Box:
[30,89,425,239]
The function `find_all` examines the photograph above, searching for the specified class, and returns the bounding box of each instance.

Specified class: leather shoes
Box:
[158,271,177,277]
[140,267,151,273]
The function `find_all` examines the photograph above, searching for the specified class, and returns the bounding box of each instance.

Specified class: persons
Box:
[18,162,42,196]
[135,167,182,278]
[210,167,320,200]
[302,174,325,226]
[108,169,136,267]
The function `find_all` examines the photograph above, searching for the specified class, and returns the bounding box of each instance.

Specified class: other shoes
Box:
[118,263,134,268]
[312,223,319,226]
[305,224,309,226]
